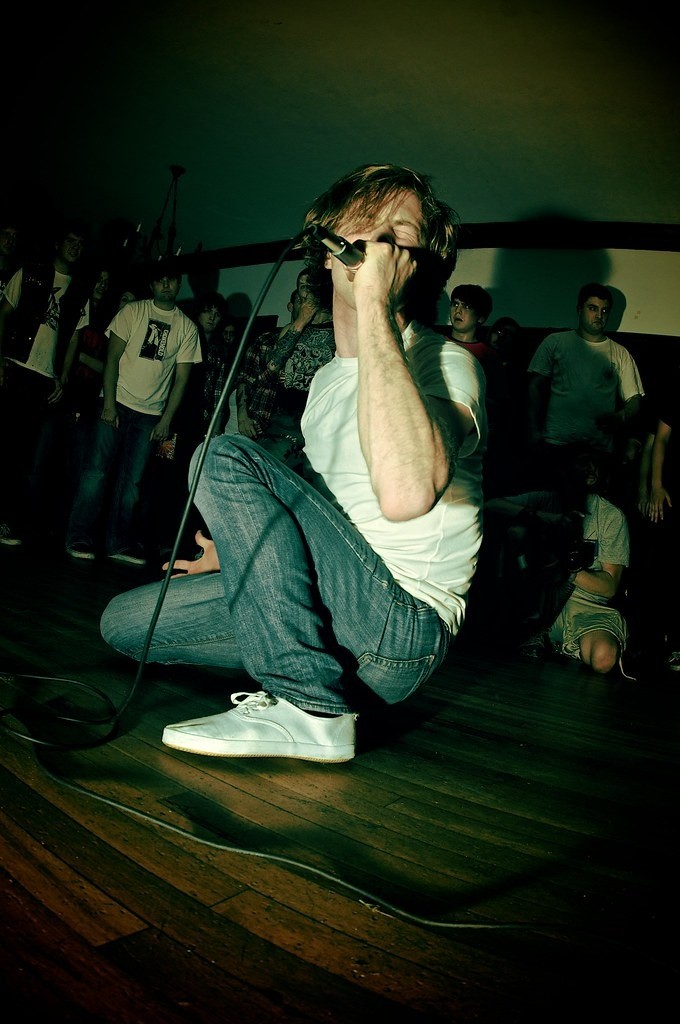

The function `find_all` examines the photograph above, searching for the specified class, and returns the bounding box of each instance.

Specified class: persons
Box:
[100,162,483,763]
[0,226,680,675]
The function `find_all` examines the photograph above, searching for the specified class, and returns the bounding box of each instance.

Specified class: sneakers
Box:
[162,691,359,763]
[108,550,146,565]
[0,525,21,546]
[664,652,680,671]
[66,545,95,559]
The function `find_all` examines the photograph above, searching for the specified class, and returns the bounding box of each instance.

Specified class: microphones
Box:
[312,224,366,275]
